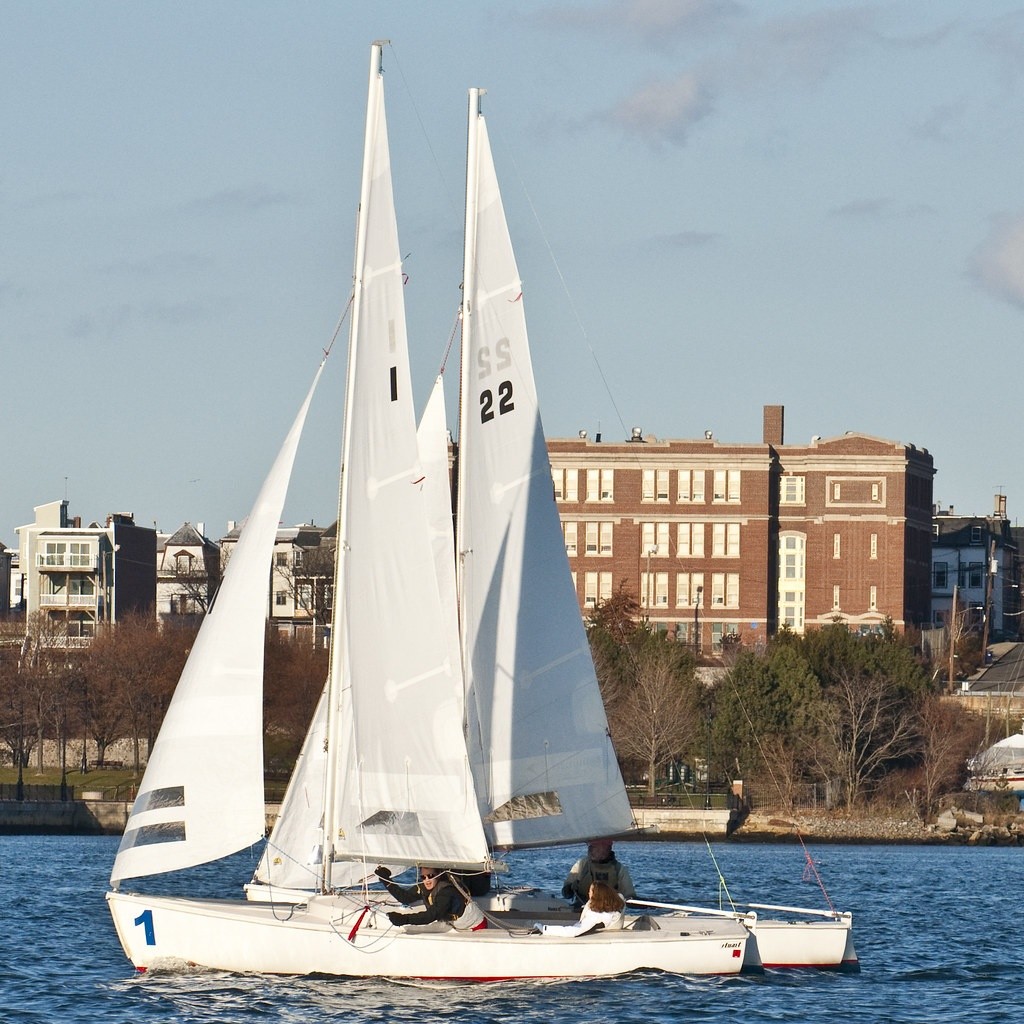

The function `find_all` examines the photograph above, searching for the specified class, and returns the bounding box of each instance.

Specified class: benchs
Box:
[89,761,123,770]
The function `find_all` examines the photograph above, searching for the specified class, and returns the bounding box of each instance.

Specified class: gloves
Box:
[375,867,391,886]
[386,912,406,926]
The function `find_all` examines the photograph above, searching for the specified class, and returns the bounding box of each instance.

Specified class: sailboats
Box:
[105,39,861,982]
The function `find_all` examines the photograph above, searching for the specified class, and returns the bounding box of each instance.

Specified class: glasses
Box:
[420,873,434,880]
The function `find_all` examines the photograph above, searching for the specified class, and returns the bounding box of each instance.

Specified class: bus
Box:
[84,758,124,773]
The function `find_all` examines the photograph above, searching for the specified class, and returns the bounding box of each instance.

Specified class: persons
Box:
[533,880,624,936]
[447,868,491,898]
[374,866,487,931]
[563,839,658,912]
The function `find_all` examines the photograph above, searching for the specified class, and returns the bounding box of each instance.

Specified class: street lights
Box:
[982,541,999,666]
[639,543,658,631]
